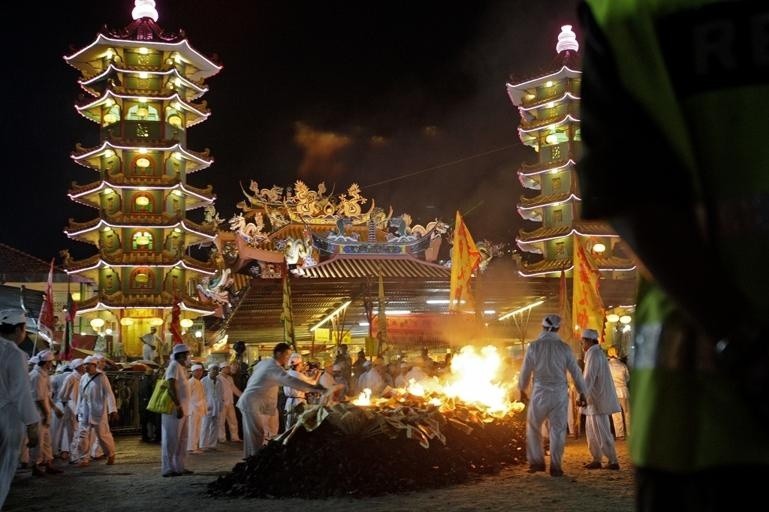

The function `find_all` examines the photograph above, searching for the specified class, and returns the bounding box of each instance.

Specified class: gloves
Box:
[26,423,41,448]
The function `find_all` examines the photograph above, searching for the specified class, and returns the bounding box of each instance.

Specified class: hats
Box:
[582,328,600,341]
[30,349,106,371]
[219,361,230,369]
[208,363,219,370]
[191,364,203,372]
[173,343,190,355]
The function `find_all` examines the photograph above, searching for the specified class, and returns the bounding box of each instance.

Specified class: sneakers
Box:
[22,451,116,478]
[162,468,194,477]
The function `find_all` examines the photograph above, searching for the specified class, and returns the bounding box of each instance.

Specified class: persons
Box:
[0,307,45,511]
[576,327,624,473]
[564,368,582,439]
[137,325,164,361]
[605,345,631,439]
[517,313,587,477]
[628,287,716,512]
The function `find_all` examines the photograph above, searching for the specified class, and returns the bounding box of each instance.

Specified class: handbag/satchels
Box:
[145,362,180,416]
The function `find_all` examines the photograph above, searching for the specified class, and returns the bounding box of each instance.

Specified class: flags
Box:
[278,257,296,348]
[37,261,55,339]
[449,208,485,316]
[571,234,608,345]
[58,292,78,362]
[557,266,572,333]
[165,293,184,350]
[376,270,390,340]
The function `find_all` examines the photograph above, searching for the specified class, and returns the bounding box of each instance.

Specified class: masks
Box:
[182,354,191,367]
